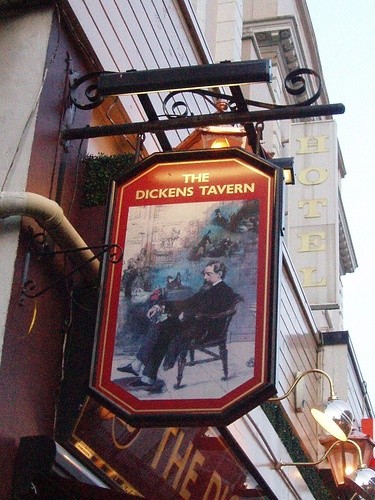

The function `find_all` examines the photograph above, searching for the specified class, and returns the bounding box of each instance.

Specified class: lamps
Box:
[266,368,354,442]
[275,440,375,500]
